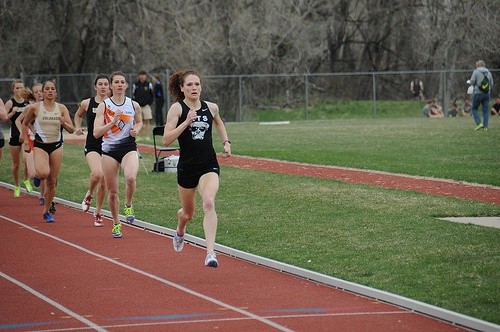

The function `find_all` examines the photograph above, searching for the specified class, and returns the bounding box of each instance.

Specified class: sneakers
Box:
[173,231,184,253]
[33,176,41,188]
[204,251,218,268]
[93,211,103,227]
[39,193,45,206]
[23,179,32,193]
[13,184,21,198]
[123,203,135,224]
[43,212,55,223]
[82,189,94,212]
[111,222,123,238]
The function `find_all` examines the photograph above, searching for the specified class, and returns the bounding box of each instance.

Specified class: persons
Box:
[74,74,110,226]
[448,104,459,117]
[5,79,33,198]
[21,80,74,221]
[15,82,55,214]
[491,97,500,115]
[152,75,165,126]
[0,98,10,161]
[163,71,231,268]
[411,77,425,101]
[470,61,493,131]
[462,98,472,117]
[132,70,154,141]
[423,99,443,118]
[93,72,143,238]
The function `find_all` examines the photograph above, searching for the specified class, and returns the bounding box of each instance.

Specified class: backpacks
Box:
[474,70,490,93]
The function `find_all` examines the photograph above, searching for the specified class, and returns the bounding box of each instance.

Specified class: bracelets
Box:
[223,139,231,145]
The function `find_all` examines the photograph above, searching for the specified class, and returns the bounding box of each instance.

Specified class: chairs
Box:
[152,125,180,172]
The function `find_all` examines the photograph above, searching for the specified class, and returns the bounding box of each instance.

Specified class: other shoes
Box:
[483,127,488,131]
[474,123,483,131]
